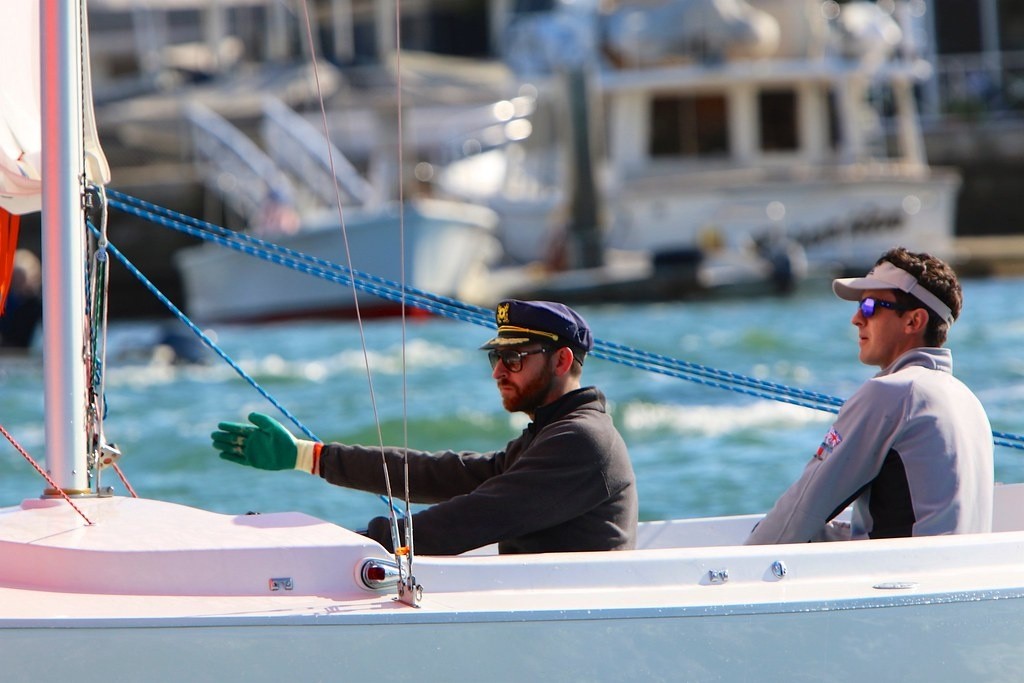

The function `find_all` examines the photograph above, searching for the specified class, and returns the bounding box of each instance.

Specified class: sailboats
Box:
[0,0,1024,681]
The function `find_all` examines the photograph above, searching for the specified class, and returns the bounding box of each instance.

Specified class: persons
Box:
[741,246,995,546]
[207,296,638,557]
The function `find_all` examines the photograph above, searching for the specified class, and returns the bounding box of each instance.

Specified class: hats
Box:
[831,261,954,329]
[478,299,594,366]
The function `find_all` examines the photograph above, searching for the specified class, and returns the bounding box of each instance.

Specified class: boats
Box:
[499,49,967,293]
[176,197,497,323]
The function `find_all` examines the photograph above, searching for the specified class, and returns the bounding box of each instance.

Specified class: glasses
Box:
[487,349,558,373]
[858,298,901,318]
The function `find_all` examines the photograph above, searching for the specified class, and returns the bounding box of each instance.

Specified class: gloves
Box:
[210,412,322,477]
